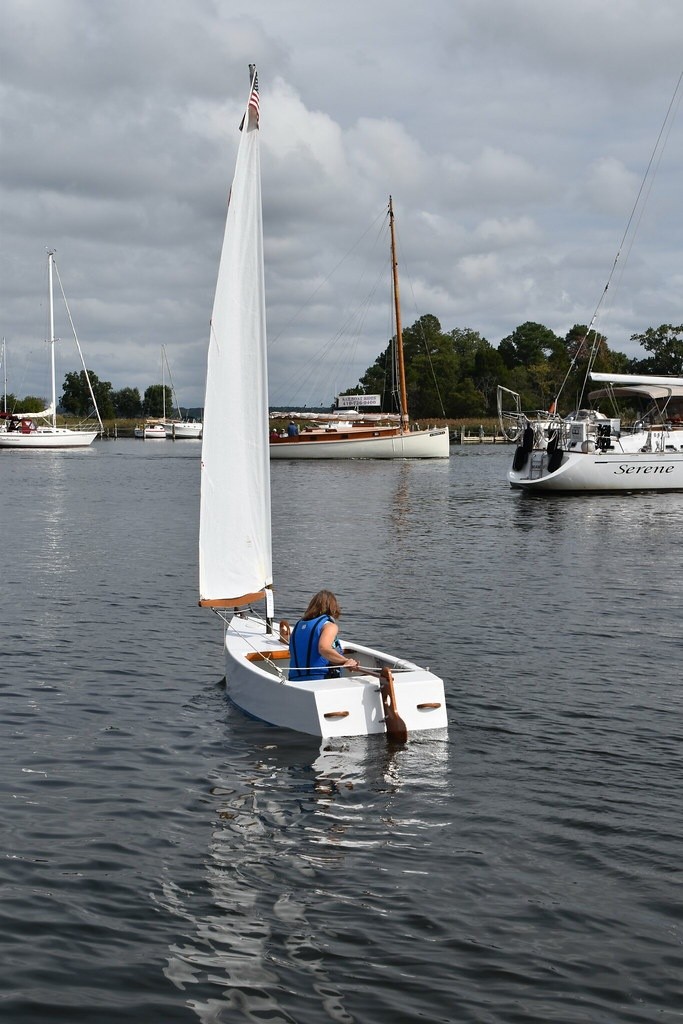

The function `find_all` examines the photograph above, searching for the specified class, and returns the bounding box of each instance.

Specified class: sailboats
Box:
[158,344,203,440]
[271,195,453,460]
[0,247,107,450]
[192,60,452,736]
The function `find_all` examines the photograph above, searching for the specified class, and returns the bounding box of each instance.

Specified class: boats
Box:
[268,418,402,443]
[498,372,683,493]
[272,401,402,422]
[137,423,167,439]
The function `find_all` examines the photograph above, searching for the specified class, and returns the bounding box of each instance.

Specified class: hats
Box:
[290,421,295,424]
[273,428,276,432]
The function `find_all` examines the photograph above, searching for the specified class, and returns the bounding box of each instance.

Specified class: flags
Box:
[238,70,258,131]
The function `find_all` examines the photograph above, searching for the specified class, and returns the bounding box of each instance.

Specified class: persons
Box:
[287,421,297,436]
[271,428,278,437]
[289,590,358,681]
[279,429,288,438]
